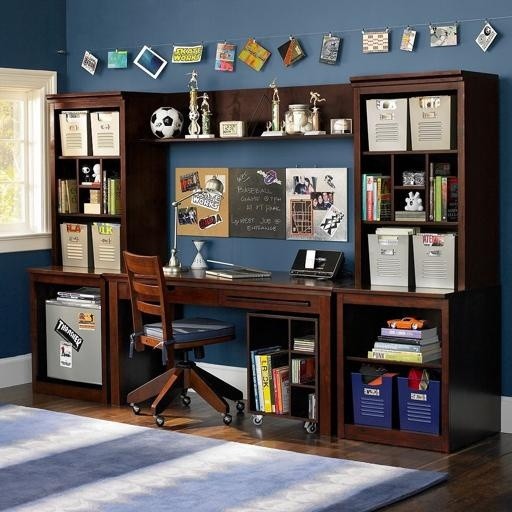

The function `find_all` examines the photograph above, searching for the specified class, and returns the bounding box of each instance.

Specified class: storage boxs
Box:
[408,95,457,150]
[394,376,442,435]
[58,112,92,156]
[92,223,122,274]
[411,231,457,289]
[365,98,410,152]
[350,373,397,429]
[59,222,93,273]
[367,234,414,292]
[43,297,102,385]
[90,110,121,156]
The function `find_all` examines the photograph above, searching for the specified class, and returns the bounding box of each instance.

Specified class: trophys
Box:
[185,68,215,139]
[304,91,326,136]
[260,80,288,137]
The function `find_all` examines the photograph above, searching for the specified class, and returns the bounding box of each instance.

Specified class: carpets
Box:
[0,404,457,512]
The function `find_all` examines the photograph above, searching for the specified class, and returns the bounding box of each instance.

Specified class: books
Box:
[368,328,442,364]
[251,338,316,419]
[103,170,120,215]
[57,287,102,304]
[58,178,78,214]
[362,162,458,223]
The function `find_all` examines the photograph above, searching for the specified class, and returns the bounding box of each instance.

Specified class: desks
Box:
[101,269,356,438]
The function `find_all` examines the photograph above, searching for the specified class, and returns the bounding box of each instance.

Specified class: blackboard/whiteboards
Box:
[229,167,286,240]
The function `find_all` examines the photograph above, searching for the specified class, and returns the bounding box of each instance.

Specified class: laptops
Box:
[206,259,271,279]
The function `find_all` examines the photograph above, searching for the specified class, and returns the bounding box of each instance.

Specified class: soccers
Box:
[151,107,184,139]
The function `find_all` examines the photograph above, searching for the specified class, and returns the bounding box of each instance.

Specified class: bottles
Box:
[282,102,315,136]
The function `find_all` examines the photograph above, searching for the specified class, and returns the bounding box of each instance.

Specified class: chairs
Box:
[122,250,245,427]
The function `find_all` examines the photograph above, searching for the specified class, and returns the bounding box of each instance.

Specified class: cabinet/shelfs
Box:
[349,71,501,294]
[25,267,110,404]
[44,90,170,273]
[333,282,502,454]
